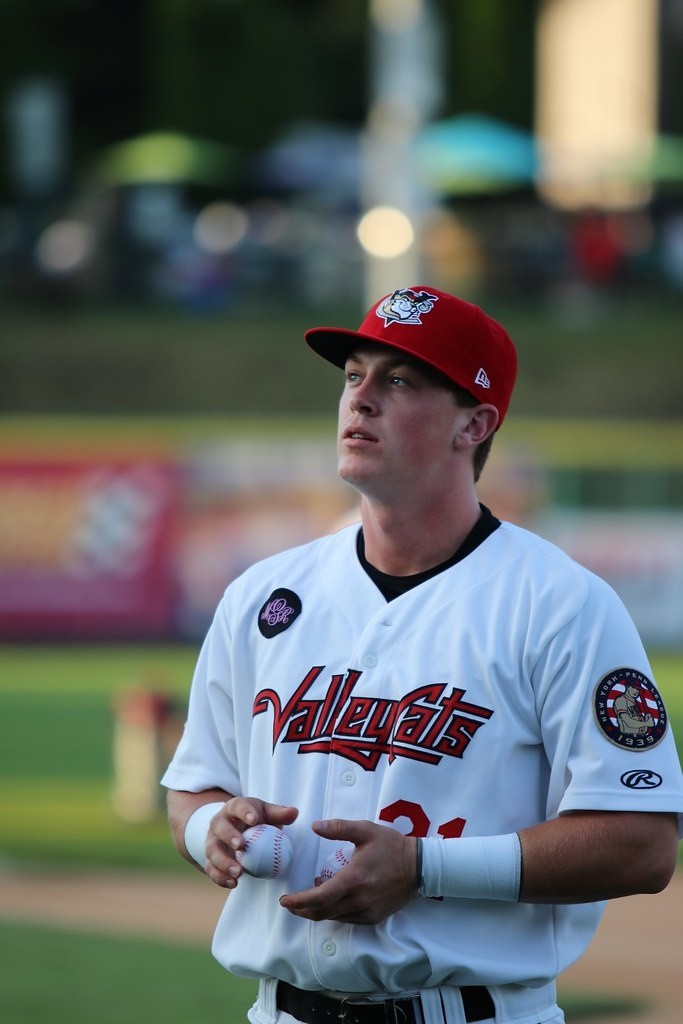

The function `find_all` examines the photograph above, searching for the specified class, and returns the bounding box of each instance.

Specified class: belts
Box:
[275,980,495,1024]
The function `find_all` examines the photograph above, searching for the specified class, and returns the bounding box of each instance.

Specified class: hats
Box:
[304,285,517,431]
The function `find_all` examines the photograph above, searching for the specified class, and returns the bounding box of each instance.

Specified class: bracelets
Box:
[416,837,422,889]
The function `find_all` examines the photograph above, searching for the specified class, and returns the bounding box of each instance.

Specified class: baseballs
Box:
[237,826,293,876]
[320,840,357,886]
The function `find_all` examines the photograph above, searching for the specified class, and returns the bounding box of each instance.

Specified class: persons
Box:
[160,285,683,1024]
